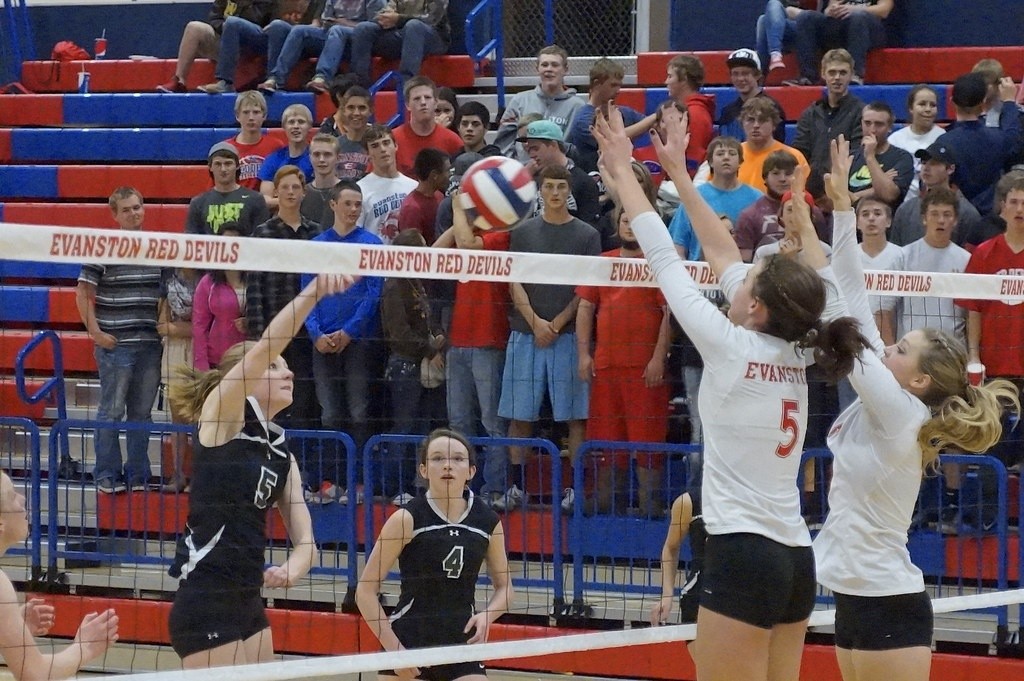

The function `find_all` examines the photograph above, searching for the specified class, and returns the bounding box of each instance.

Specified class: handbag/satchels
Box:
[420,345,446,388]
[51,42,91,60]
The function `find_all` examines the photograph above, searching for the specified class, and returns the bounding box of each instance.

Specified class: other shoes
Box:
[905,514,928,535]
[342,481,367,505]
[98,477,126,493]
[393,493,415,505]
[782,77,814,86]
[769,51,786,70]
[160,472,187,493]
[304,489,335,504]
[131,476,145,491]
[937,504,962,537]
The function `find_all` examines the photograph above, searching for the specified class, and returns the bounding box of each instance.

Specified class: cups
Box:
[77,72,90,93]
[94,38,106,59]
[966,362,986,387]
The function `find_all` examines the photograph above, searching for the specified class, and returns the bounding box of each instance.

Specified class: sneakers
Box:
[492,485,529,511]
[562,487,574,513]
[198,79,236,93]
[156,77,188,93]
[968,517,997,538]
[306,77,328,92]
[257,77,286,92]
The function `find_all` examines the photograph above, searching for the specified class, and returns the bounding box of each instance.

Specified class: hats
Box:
[208,142,241,178]
[915,143,954,162]
[952,73,987,106]
[515,120,563,142]
[727,48,761,72]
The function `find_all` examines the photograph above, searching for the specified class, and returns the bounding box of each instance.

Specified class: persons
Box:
[585,94,877,681]
[257,0,366,93]
[165,249,323,672]
[780,0,896,86]
[155,0,272,91]
[491,164,601,512]
[350,0,453,87]
[575,203,674,519]
[72,186,167,495]
[157,46,1024,527]
[352,428,515,681]
[789,127,1021,681]
[195,0,315,93]
[0,468,120,681]
[753,0,829,85]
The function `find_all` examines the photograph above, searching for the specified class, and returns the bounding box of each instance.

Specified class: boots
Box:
[584,464,622,515]
[636,466,667,520]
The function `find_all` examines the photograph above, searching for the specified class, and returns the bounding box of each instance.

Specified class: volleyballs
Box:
[457,155,538,233]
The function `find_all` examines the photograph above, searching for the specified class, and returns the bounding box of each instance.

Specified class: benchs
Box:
[0,46,1024,582]
